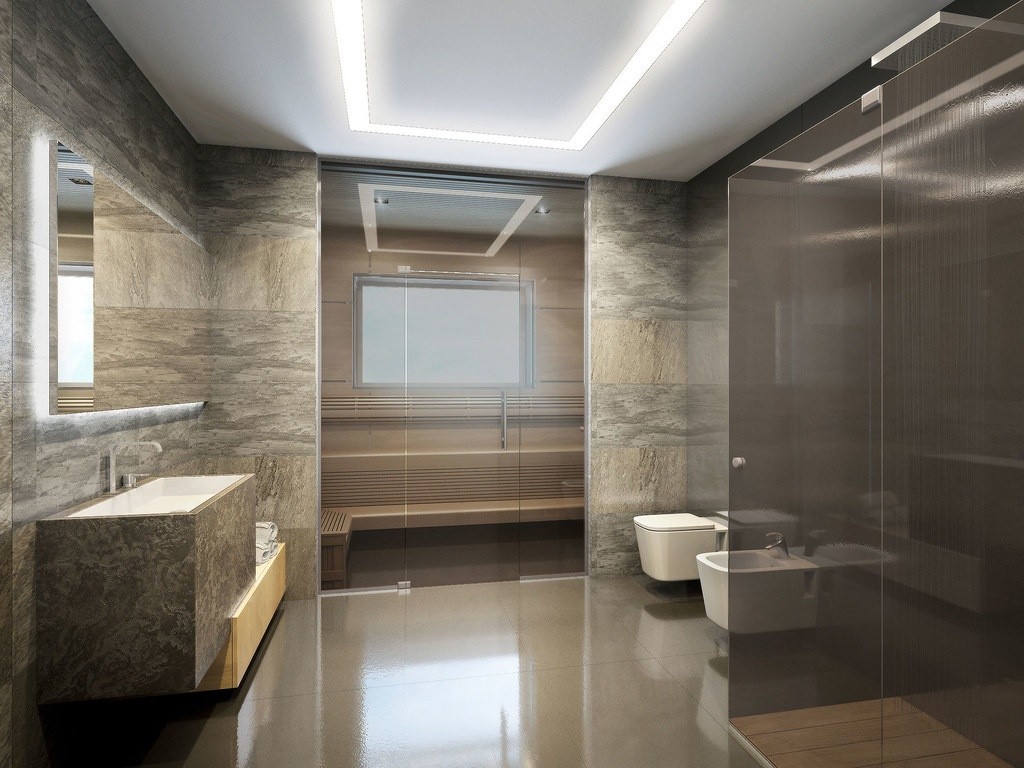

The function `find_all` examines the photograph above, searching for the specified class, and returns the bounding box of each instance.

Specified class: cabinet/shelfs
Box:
[193,543,288,692]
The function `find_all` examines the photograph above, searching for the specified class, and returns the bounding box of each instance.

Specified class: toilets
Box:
[633,512,716,581]
[716,508,800,549]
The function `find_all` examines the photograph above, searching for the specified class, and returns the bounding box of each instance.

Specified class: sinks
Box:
[696,549,819,635]
[66,474,247,518]
[788,543,897,627]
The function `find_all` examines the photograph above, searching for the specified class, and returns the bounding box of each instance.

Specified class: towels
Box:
[255,522,279,564]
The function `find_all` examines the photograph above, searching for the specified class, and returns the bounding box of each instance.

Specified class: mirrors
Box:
[48,139,96,383]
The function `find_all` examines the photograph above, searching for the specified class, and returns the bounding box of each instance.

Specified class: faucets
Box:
[109,441,163,493]
[804,530,833,556]
[765,533,790,560]
